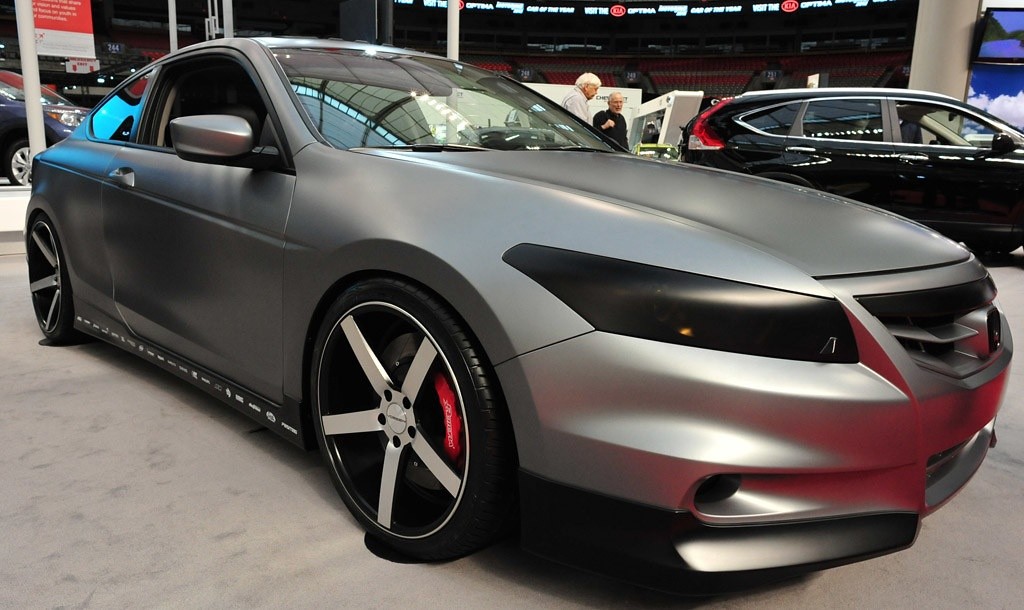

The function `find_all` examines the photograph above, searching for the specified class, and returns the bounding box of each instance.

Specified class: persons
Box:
[560,72,601,127]
[592,92,629,153]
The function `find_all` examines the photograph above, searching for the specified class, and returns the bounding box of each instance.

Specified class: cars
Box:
[23,34,1014,600]
[0,68,96,187]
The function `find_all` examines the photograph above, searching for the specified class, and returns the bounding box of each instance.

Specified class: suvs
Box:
[678,89,1024,261]
